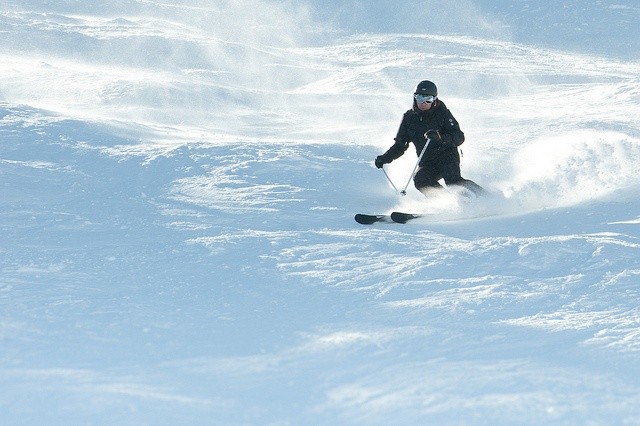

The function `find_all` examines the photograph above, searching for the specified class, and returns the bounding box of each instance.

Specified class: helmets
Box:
[414,80,437,97]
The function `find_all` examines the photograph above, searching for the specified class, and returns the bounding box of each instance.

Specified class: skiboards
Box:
[355,211,421,226]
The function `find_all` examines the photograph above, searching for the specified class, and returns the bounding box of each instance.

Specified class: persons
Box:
[376,80,497,200]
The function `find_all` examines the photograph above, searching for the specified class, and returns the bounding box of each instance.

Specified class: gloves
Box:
[375,154,393,169]
[424,129,443,143]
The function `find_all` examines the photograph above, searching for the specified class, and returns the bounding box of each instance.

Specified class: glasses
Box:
[415,94,437,104]
